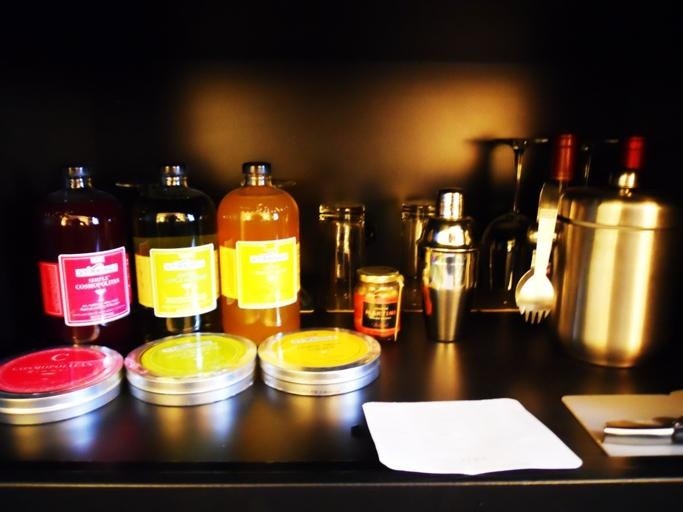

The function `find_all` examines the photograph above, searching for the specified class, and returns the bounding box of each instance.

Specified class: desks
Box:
[0,312,681,511]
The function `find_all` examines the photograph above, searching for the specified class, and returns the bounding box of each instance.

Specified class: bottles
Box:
[132,165,220,341]
[35,163,130,347]
[214,160,301,344]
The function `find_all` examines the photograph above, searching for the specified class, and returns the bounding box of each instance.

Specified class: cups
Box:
[314,202,365,310]
[479,139,548,310]
[398,199,439,311]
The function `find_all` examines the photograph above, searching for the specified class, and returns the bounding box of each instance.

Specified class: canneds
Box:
[354,266,403,340]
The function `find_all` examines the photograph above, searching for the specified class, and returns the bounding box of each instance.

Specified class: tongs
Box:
[515,178,564,326]
